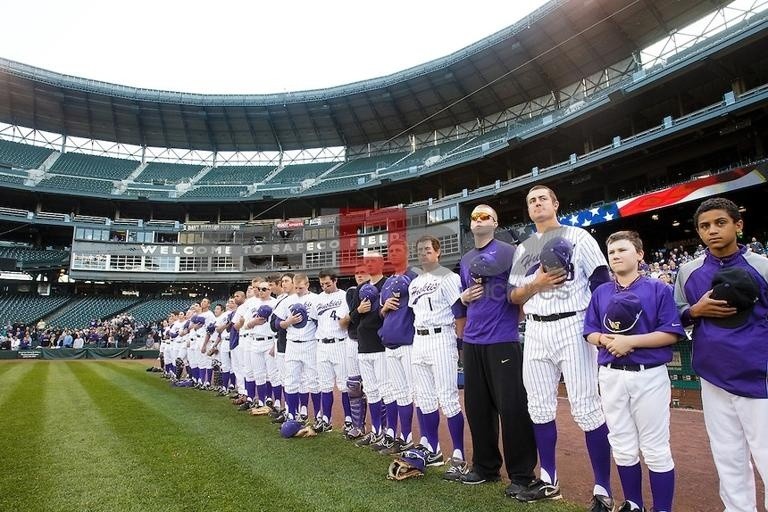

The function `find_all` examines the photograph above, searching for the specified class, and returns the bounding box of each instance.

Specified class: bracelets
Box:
[597,333,602,346]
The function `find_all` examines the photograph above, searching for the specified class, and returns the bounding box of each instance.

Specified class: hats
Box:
[604,292,643,335]
[359,284,379,312]
[291,303,307,328]
[184,315,205,333]
[710,267,760,329]
[469,253,497,284]
[381,274,411,307]
[258,305,272,317]
[207,321,216,334]
[540,236,572,284]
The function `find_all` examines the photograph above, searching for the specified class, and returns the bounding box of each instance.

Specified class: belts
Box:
[318,338,344,343]
[417,328,441,335]
[527,311,576,321]
[253,337,272,341]
[600,360,655,372]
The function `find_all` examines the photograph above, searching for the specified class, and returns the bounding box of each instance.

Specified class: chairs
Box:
[1,108,556,198]
[0,246,233,345]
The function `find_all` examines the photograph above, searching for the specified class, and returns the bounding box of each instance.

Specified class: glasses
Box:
[471,212,496,222]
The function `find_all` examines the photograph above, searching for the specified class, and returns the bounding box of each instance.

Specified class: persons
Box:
[159,235,164,242]
[159,262,369,441]
[671,196,768,512]
[372,240,422,454]
[0,311,162,350]
[111,233,118,242]
[507,182,615,511]
[622,234,768,308]
[458,203,535,498]
[347,251,398,453]
[120,235,125,241]
[583,231,687,512]
[405,237,465,480]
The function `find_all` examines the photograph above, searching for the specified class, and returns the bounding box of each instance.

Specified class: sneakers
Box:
[344,421,562,502]
[617,501,646,512]
[589,495,614,512]
[160,372,334,432]
